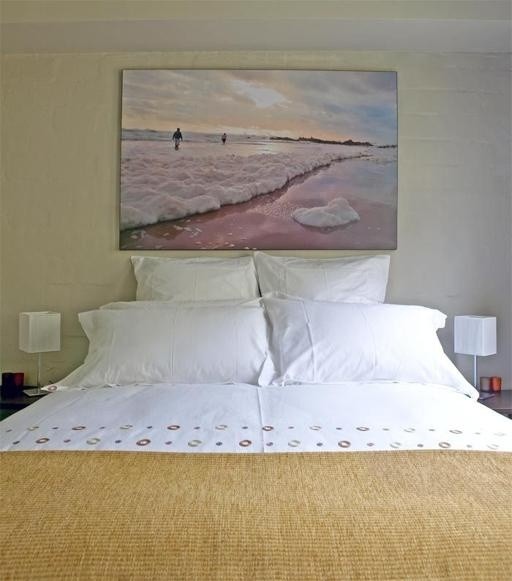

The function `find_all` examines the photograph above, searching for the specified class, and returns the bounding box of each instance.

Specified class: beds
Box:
[0,380,512,581]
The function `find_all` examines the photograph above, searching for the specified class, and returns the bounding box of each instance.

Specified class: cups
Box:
[14,372,24,388]
[480,377,490,393]
[2,372,14,389]
[490,376,501,393]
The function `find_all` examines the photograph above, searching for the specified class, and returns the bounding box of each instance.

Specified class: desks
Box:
[0,384,51,410]
[477,389,512,418]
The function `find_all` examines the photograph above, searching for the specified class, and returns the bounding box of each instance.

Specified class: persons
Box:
[221,133,227,146]
[172,128,183,151]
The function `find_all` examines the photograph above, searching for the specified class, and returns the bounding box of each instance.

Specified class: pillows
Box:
[253,251,392,304]
[257,292,448,389]
[129,254,260,301]
[83,296,262,363]
[62,305,268,390]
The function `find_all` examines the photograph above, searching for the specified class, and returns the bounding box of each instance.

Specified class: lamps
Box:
[17,310,63,398]
[452,314,500,400]
